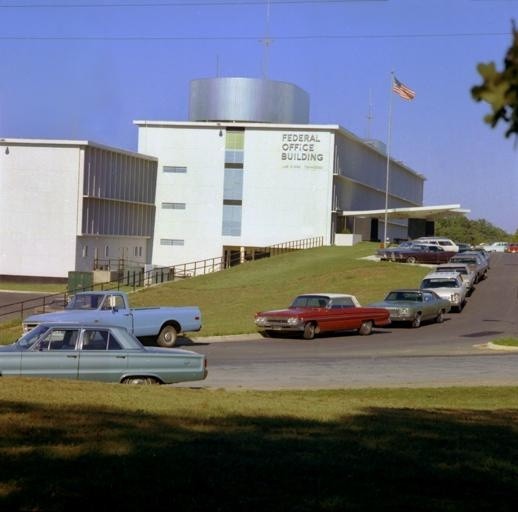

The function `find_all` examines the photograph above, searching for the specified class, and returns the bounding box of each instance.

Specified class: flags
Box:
[393,74,416,101]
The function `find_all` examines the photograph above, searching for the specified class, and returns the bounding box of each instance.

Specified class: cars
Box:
[377,234,490,312]
[368,289,452,328]
[254,293,392,338]
[0,323,208,386]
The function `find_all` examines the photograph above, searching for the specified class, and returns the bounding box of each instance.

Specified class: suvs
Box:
[470,241,517,254]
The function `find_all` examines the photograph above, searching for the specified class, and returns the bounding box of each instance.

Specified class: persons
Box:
[385,237,391,248]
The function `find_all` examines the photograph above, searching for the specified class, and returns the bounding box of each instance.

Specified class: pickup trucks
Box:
[22,291,202,346]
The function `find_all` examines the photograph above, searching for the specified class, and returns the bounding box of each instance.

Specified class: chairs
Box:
[318,299,326,307]
[91,296,99,309]
[395,293,405,300]
[62,331,72,349]
